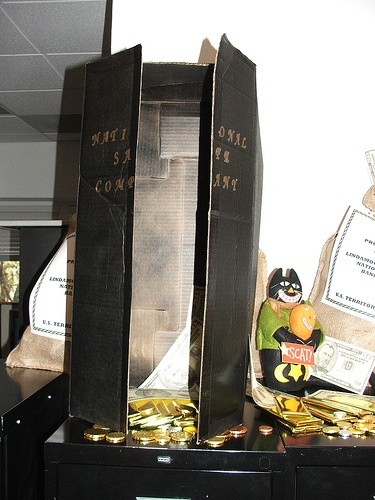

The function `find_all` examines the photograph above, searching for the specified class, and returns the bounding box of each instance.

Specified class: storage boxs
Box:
[67,31,265,446]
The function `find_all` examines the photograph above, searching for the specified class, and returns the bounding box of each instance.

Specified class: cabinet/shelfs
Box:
[43,417,293,500]
[269,374,375,500]
